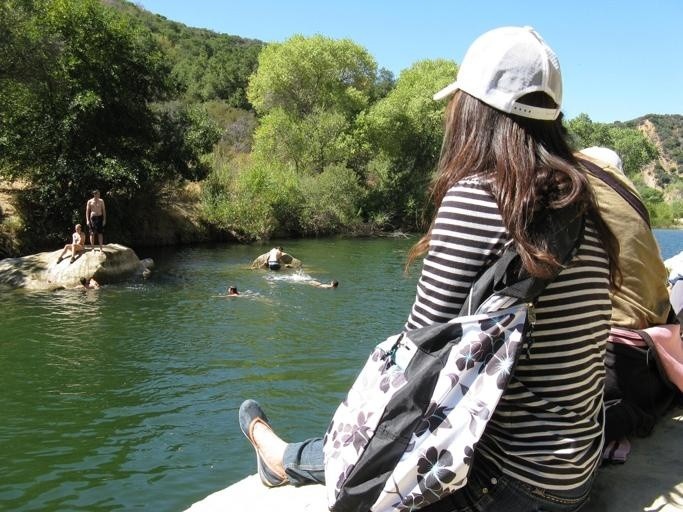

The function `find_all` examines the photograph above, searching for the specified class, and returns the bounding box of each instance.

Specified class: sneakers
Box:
[238,398,291,488]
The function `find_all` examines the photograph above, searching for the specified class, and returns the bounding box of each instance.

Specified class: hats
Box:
[431,26,563,122]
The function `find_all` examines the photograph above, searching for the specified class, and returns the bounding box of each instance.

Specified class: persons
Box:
[567,146,683,455]
[265,246,284,272]
[85,189,108,256]
[226,285,241,297]
[307,278,339,289]
[56,223,86,264]
[235,23,622,512]
